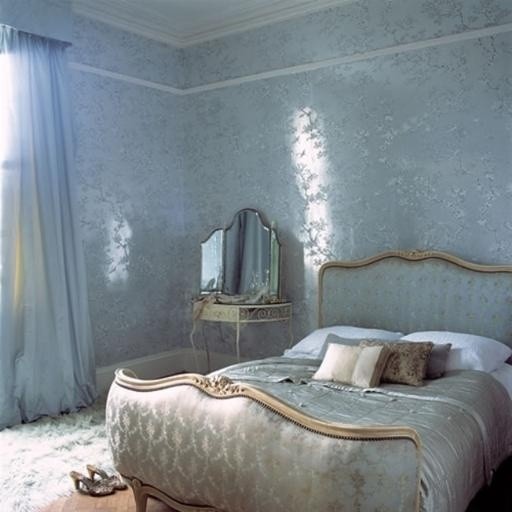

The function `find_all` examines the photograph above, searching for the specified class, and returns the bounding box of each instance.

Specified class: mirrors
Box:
[200,208,283,300]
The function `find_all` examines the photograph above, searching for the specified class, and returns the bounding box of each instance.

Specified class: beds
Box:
[106,248,512,512]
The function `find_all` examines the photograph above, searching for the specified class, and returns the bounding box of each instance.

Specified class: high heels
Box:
[69,469,115,497]
[83,463,127,490]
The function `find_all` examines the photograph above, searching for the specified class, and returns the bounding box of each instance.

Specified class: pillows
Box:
[282,325,512,389]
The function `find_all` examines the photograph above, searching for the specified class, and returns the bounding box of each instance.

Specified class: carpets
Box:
[0,394,122,512]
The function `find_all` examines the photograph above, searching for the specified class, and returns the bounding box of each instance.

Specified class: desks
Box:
[190,300,294,374]
